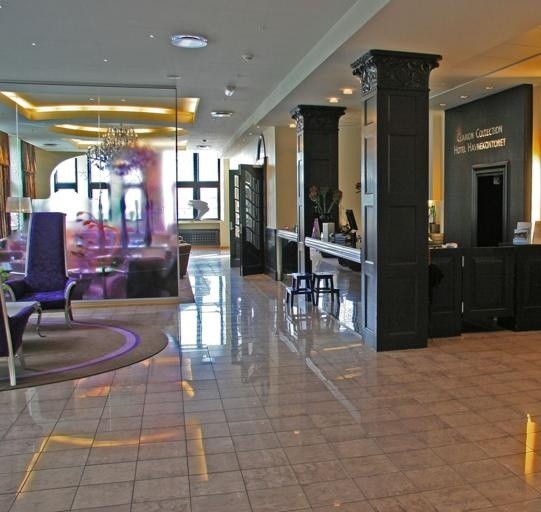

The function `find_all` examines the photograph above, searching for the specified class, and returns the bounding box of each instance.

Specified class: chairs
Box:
[124,229,192,297]
[1,212,78,386]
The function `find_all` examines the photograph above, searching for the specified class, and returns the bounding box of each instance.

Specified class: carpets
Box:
[0,317,168,392]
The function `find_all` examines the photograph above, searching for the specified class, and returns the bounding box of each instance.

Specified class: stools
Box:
[315,271,341,306]
[285,273,315,308]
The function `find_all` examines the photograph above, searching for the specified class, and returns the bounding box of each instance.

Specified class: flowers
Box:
[308,185,343,214]
[428,199,437,223]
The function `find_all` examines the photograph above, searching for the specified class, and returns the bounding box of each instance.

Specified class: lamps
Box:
[6,197,32,239]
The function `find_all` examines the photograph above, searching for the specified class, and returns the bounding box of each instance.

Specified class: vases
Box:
[430,222,440,233]
[319,213,333,232]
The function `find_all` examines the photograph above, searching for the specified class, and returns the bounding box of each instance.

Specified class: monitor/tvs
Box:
[344,209,359,234]
